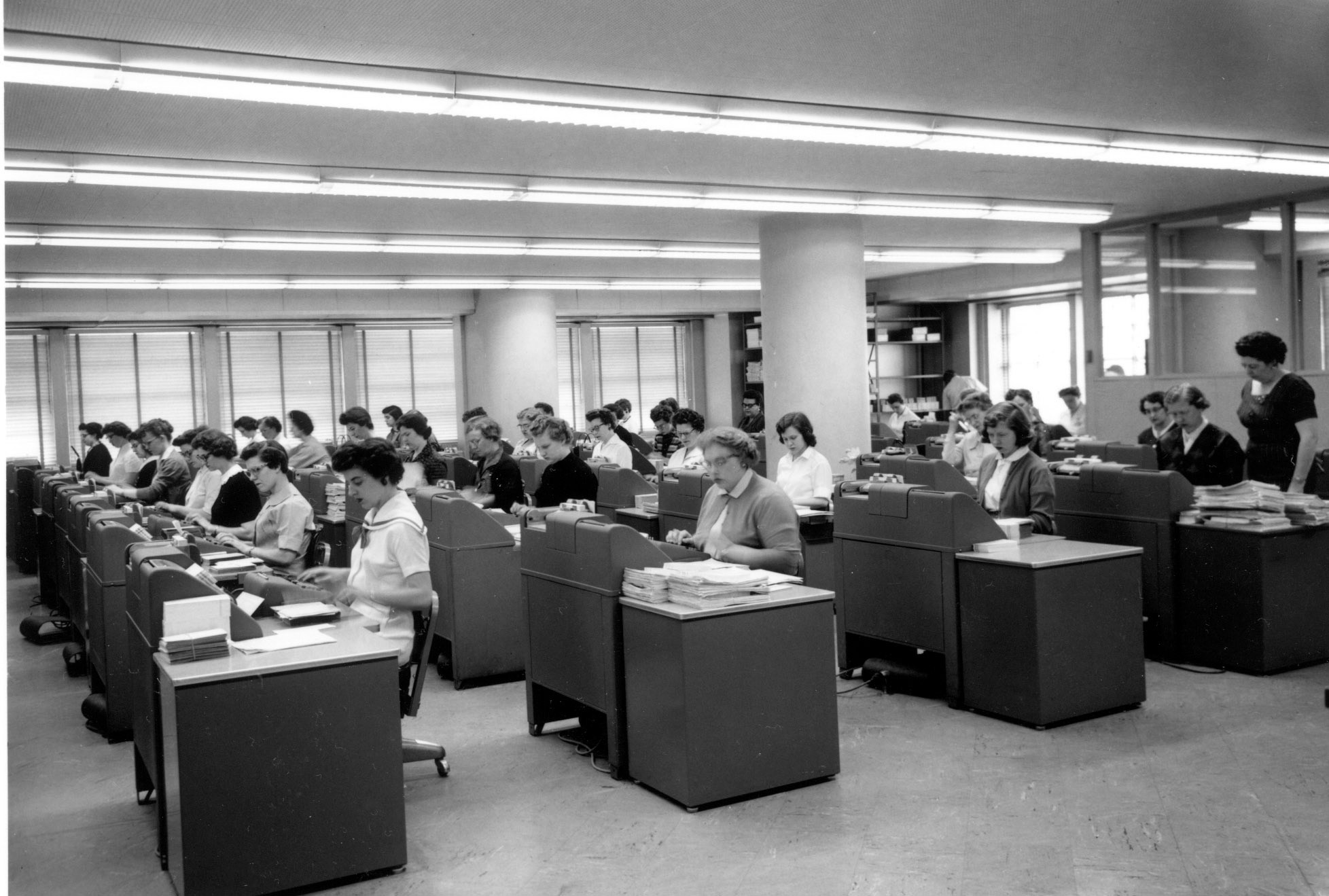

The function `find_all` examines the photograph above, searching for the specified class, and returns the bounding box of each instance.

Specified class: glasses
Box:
[677,428,695,438]
[1144,407,1163,414]
[142,437,159,445]
[178,449,193,458]
[653,423,668,429]
[244,464,268,477]
[741,402,755,408]
[104,433,117,440]
[590,423,604,432]
[130,443,141,449]
[195,452,212,462]
[468,439,480,446]
[79,433,90,439]
[965,414,979,424]
[701,453,738,470]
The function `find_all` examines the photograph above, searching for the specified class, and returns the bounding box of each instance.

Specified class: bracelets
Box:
[249,546,255,557]
[214,525,220,535]
[1292,475,1306,484]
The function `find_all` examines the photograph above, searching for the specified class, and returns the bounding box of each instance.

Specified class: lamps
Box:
[0,31,1329,292]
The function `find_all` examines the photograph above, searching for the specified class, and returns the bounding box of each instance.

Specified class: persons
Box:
[1059,385,1086,436]
[1152,382,1245,487]
[774,412,834,545]
[738,390,765,433]
[585,408,633,469]
[76,368,1246,719]
[184,440,317,576]
[976,401,1058,536]
[461,416,524,514]
[941,391,998,478]
[296,437,432,719]
[887,392,921,441]
[668,408,708,469]
[1234,331,1319,494]
[102,418,192,519]
[511,415,599,520]
[665,426,805,577]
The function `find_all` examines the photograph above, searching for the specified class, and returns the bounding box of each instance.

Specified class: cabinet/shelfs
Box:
[1175,518,1329,678]
[955,540,1148,730]
[615,508,660,543]
[619,585,841,813]
[153,619,408,896]
[201,554,273,596]
[315,514,347,568]
[740,311,946,439]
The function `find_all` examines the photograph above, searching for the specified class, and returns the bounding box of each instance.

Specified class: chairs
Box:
[398,589,451,776]
